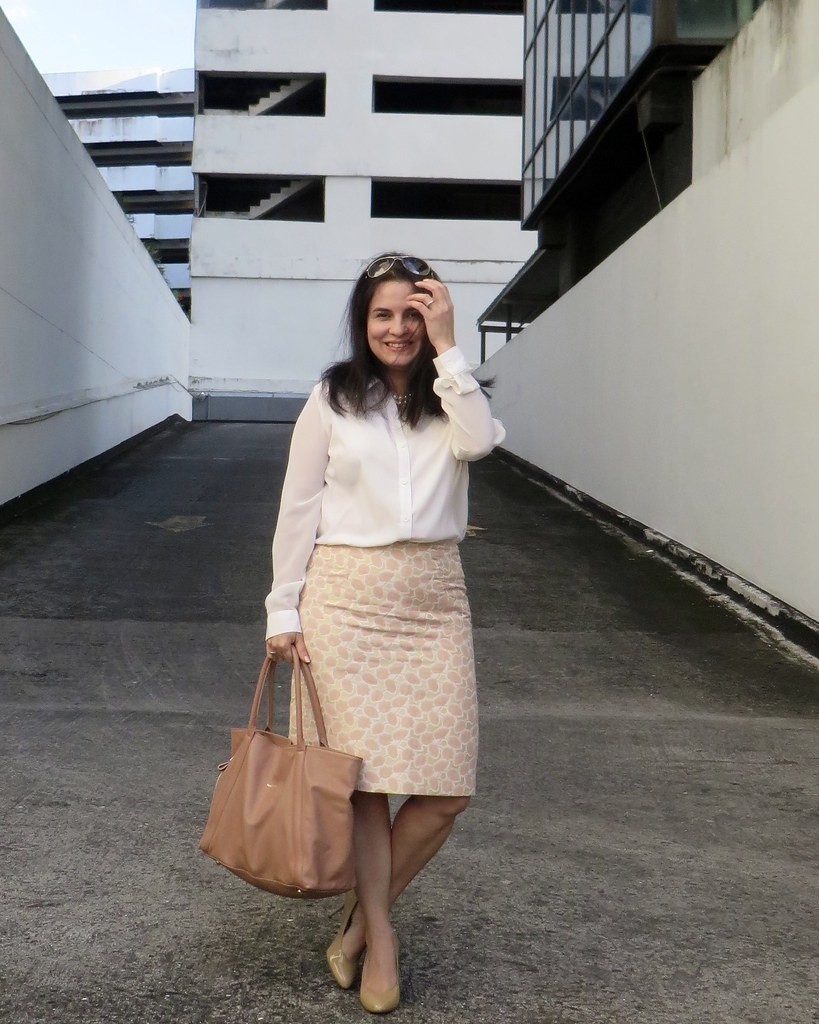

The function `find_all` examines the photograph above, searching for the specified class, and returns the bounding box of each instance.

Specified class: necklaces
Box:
[391,394,412,404]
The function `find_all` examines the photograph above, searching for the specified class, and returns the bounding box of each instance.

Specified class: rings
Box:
[270,651,276,654]
[426,300,433,307]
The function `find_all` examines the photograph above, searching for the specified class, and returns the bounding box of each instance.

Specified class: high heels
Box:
[324,890,366,991]
[359,924,401,1014]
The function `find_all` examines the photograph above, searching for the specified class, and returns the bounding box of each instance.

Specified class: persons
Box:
[265,253,507,1014]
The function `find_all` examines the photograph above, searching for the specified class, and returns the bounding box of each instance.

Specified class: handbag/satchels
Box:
[198,642,363,899]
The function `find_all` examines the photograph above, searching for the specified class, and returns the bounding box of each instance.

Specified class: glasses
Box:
[364,255,434,282]
